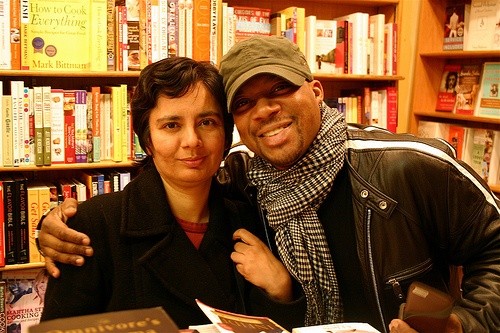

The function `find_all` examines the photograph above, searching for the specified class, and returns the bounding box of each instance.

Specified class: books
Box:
[0,0,500,333]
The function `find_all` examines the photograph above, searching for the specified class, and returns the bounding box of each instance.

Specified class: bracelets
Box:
[36,205,58,256]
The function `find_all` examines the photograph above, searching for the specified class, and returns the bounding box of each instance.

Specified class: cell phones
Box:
[402,281,454,333]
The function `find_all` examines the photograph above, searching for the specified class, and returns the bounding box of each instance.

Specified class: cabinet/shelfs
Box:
[0,0,500,270]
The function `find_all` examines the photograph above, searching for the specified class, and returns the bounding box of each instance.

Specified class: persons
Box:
[9,268,48,309]
[38,35,500,333]
[442,72,457,93]
[42,56,307,333]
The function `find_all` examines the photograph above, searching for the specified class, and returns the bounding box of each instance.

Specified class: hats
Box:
[218,35,313,114]
